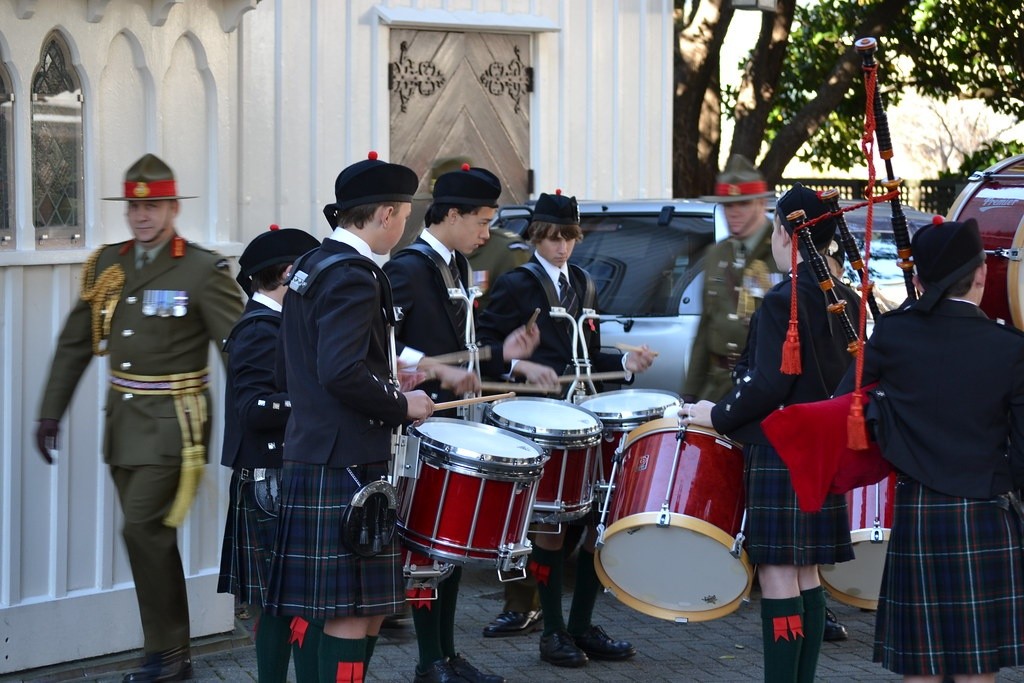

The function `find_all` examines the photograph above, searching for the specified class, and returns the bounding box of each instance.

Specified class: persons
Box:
[263,150,435,683]
[831,215,1024,683]
[217,224,321,683]
[678,155,868,683]
[381,162,657,683]
[34,155,249,683]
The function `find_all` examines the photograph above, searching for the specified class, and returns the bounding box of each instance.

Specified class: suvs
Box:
[465,197,945,590]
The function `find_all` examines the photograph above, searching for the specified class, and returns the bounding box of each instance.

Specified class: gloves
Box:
[36,419,60,464]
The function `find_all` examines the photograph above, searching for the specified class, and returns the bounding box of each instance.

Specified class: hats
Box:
[424,162,502,228]
[697,153,776,201]
[829,233,846,267]
[776,183,837,247]
[323,151,420,232]
[531,188,581,225]
[98,153,200,201]
[236,223,322,299]
[908,215,988,314]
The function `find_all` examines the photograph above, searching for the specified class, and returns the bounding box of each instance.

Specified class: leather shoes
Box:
[483,610,545,637]
[823,604,850,640]
[569,625,637,660]
[413,656,471,683]
[123,654,193,683]
[538,633,589,667]
[450,653,506,683]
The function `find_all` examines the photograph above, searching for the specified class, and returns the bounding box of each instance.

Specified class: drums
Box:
[594,419,753,626]
[395,416,549,570]
[945,153,1024,332]
[580,388,681,485]
[817,473,898,612]
[482,395,605,522]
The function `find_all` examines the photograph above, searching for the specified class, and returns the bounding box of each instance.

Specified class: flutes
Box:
[784,38,920,357]
